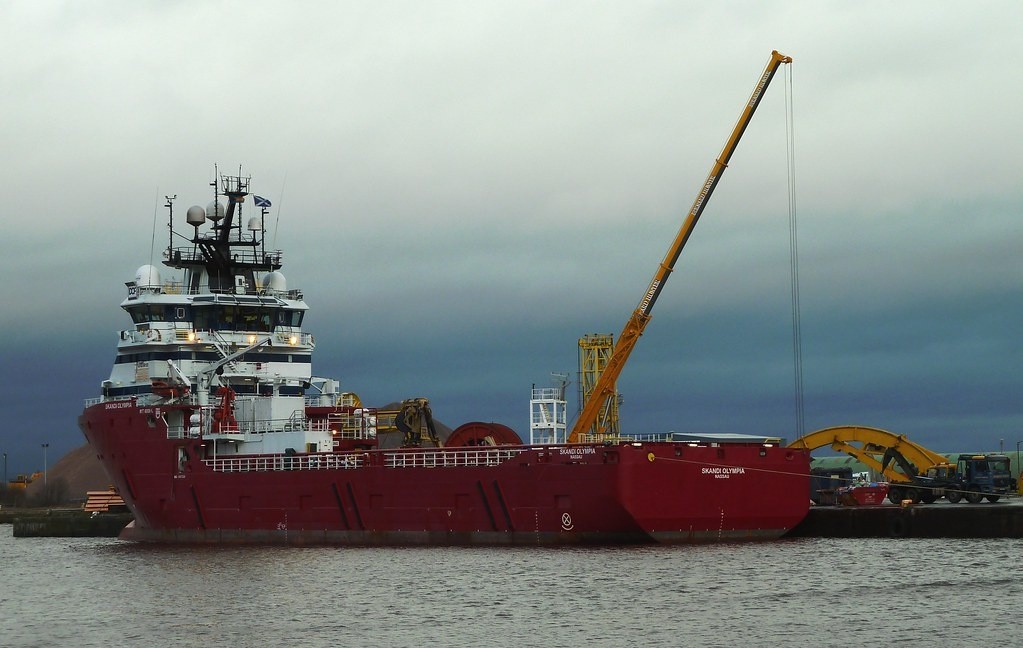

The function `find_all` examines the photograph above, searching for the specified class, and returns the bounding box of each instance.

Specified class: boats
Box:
[78,162,811,545]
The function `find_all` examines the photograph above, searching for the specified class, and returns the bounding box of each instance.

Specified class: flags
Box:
[253,195,271,207]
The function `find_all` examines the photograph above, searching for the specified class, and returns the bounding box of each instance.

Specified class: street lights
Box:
[42,442,49,486]
[3,452,6,489]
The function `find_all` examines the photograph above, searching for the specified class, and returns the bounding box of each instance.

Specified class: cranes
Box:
[564,50,806,445]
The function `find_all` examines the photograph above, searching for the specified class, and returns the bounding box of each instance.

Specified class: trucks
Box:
[887,454,1012,504]
